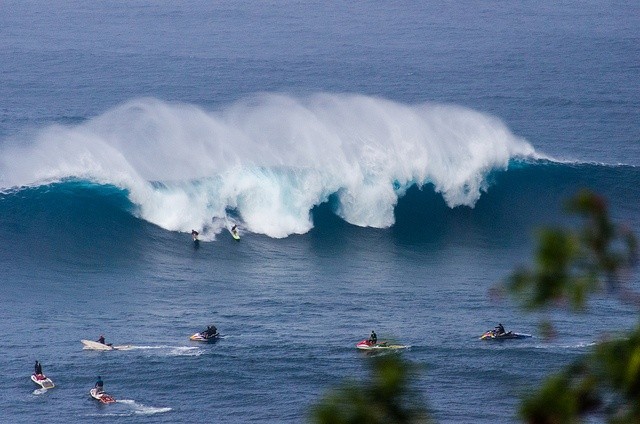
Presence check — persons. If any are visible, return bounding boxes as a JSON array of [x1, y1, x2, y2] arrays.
[[496, 323, 504, 339], [97, 336, 106, 343], [94, 376, 108, 387], [369, 330, 378, 345], [202, 326, 212, 338], [34, 360, 43, 373]]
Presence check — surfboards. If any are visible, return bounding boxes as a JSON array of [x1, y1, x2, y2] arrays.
[[230, 229, 241, 240]]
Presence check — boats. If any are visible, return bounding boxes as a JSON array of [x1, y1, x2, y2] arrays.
[[190, 332, 218, 341], [31, 374, 55, 390], [356, 339, 411, 350], [90, 388, 116, 404], [193, 235, 200, 242], [227, 223, 240, 239], [479, 329, 532, 340]]
